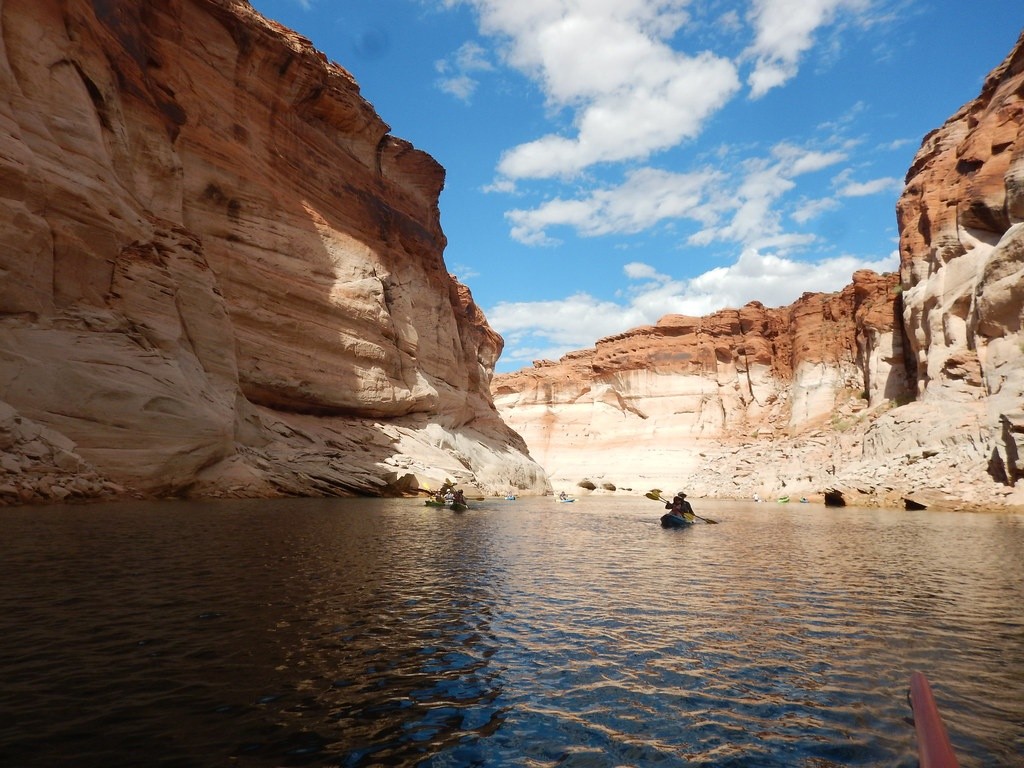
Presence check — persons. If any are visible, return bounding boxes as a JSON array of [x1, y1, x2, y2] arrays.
[[433, 487, 467, 506], [560, 491, 566, 500], [666, 492, 695, 517], [753, 492, 759, 502]]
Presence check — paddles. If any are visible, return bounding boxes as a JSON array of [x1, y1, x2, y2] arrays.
[[652, 490, 694, 521], [646, 493, 717, 524]]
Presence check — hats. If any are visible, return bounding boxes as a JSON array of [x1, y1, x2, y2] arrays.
[[678, 492, 686, 497], [447, 489, 450, 491], [674, 496, 682, 498]]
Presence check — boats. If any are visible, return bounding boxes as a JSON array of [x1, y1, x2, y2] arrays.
[[778, 495, 790, 503], [425, 500, 466, 511], [555, 498, 575, 503], [504, 496, 515, 501], [659, 513, 696, 528], [799, 498, 809, 503]]
